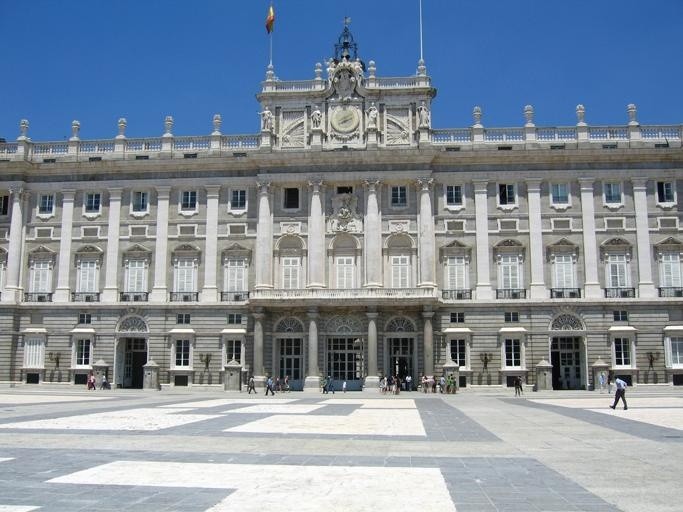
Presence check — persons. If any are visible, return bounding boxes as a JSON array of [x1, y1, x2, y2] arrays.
[[514, 376, 521, 397], [378, 373, 456, 395], [265, 376, 275, 396], [416, 101, 430, 127], [248, 376, 258, 394], [284, 376, 290, 392], [309, 105, 321, 128], [88, 373, 96, 390], [275, 376, 282, 393], [258, 107, 272, 129], [610, 376, 627, 410], [320, 375, 334, 394], [364, 101, 378, 127], [343, 379, 347, 393], [100, 372, 108, 390]]
